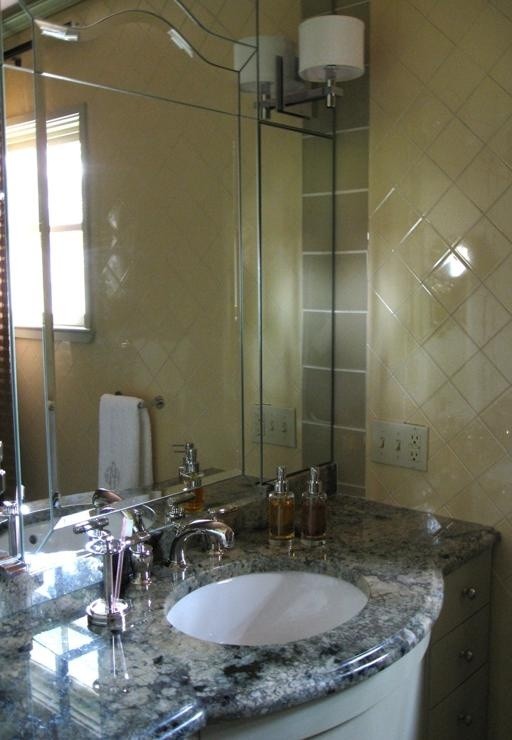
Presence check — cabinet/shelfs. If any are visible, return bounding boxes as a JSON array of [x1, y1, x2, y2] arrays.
[[422, 549, 496, 739]]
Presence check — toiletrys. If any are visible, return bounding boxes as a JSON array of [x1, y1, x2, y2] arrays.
[[300, 466, 328, 540], [174, 442, 204, 511], [267, 467, 296, 539]]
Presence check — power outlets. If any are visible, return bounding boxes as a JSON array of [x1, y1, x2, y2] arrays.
[[401, 422, 430, 472]]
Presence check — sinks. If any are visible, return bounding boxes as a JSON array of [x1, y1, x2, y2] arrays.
[[163, 559, 371, 647], [0, 502, 157, 555]]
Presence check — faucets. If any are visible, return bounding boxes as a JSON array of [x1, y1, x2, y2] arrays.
[[169, 519, 234, 565], [91, 488, 145, 534]]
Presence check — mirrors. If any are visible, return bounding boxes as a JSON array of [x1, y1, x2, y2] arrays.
[[1, 0, 340, 577]]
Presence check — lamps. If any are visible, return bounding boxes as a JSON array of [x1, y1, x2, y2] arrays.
[[233, 32, 281, 122], [282, 13, 366, 114]]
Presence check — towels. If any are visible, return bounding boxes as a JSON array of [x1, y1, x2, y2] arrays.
[[96, 393, 156, 493]]
